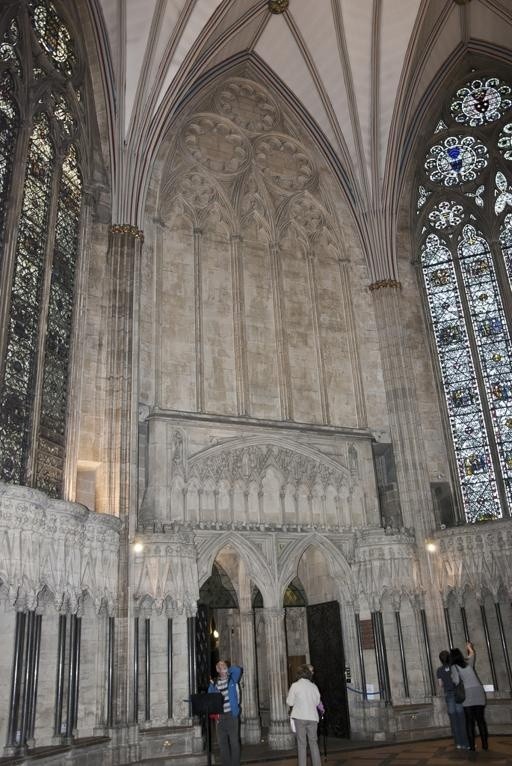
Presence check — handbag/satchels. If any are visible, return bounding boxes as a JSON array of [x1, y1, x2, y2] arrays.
[[453, 680, 466, 705]]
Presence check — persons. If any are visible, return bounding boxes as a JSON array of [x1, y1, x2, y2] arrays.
[[448, 640, 489, 752], [287, 665, 323, 766], [434, 650, 472, 752], [307, 665, 331, 764], [207, 661, 243, 766]]
